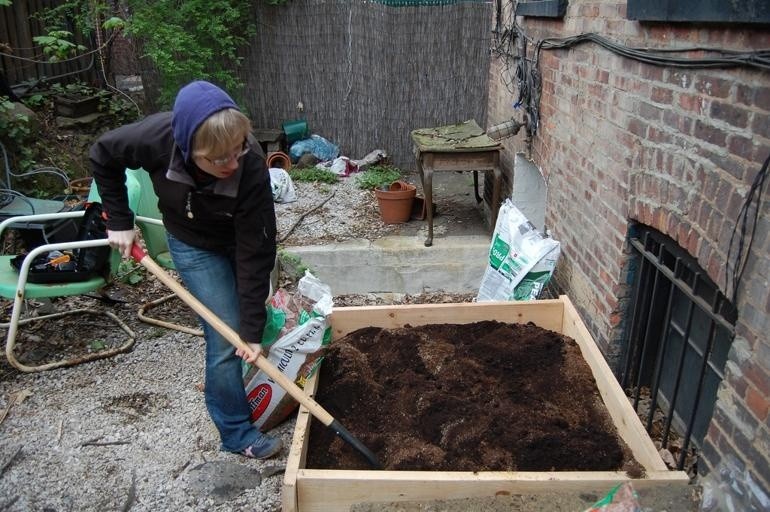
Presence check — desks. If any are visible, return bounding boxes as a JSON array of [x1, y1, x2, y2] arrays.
[[411, 124, 508, 248]]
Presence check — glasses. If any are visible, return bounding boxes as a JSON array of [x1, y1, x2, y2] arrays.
[[204, 141, 252, 166]]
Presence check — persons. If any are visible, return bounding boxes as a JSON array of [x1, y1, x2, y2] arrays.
[[87, 79, 284, 460]]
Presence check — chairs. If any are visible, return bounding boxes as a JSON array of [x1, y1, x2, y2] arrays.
[[125, 168, 204, 335], [0, 143, 78, 255], [1, 171, 141, 373]]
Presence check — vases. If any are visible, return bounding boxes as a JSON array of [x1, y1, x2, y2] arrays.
[[375, 184, 416, 225]]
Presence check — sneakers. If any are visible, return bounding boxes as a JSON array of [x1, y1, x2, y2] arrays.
[[239, 434, 283, 459]]
